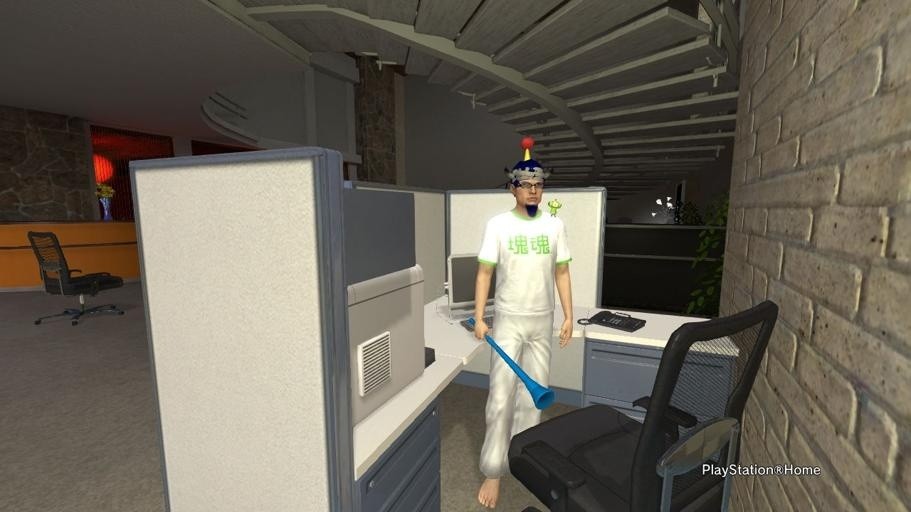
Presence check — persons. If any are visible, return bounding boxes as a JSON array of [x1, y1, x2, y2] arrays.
[[472, 139, 576, 510]]
